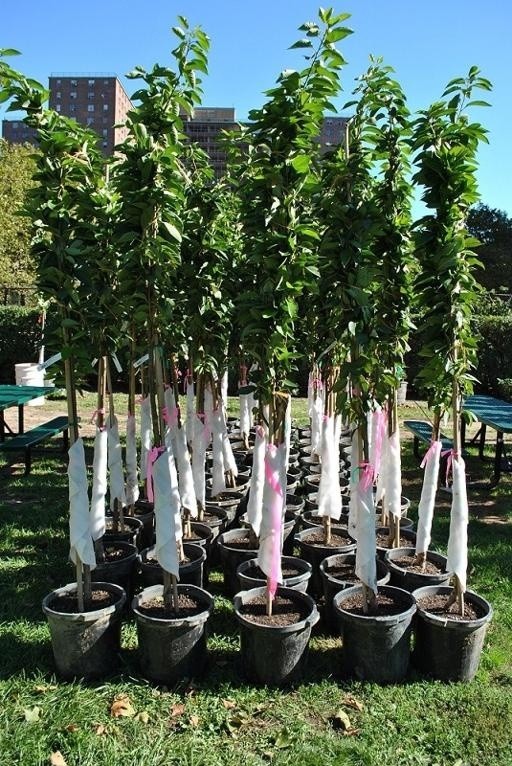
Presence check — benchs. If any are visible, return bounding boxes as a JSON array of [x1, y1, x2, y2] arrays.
[[404, 419, 470, 469], [1, 415, 81, 476]]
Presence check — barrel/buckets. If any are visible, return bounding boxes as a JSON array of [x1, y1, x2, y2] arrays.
[[14, 362, 56, 405]]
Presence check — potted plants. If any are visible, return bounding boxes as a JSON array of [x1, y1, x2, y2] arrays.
[[14, 4, 493, 683]]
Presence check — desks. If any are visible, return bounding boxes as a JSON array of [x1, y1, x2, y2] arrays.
[[1, 384, 57, 440], [461, 395, 512, 475]]
